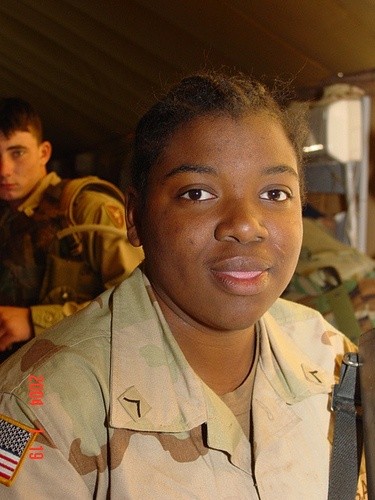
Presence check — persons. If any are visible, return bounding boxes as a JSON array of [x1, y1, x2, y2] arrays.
[[0, 74, 375, 500]]
